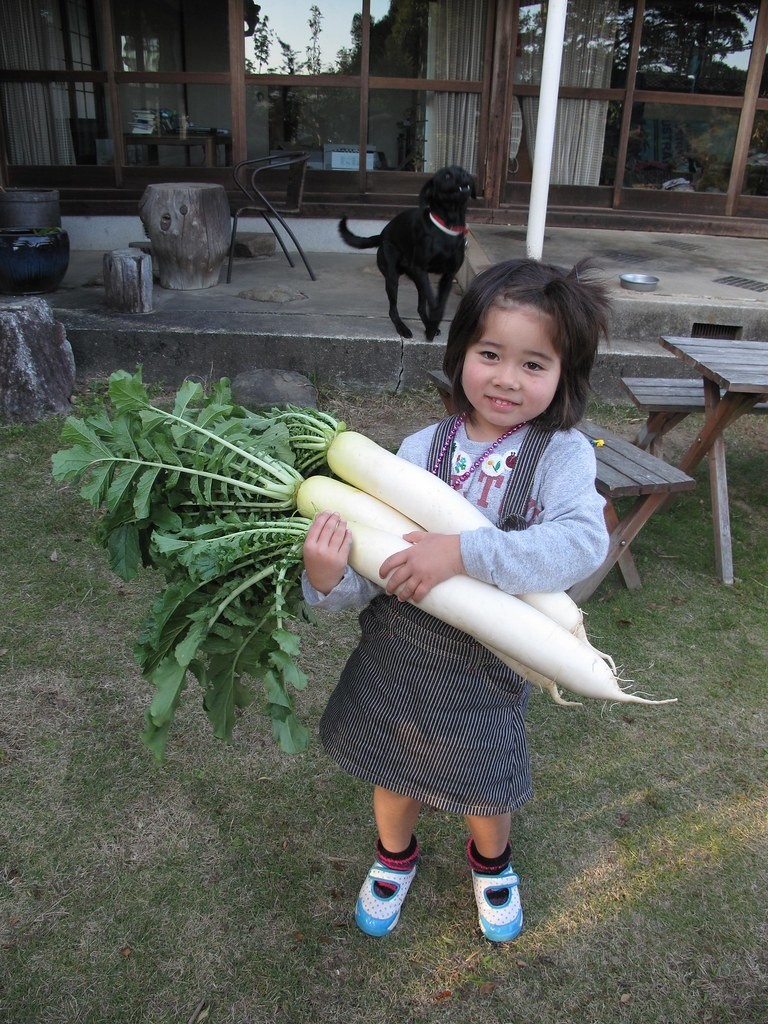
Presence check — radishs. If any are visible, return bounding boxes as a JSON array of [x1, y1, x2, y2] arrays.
[[296, 430, 680, 706]]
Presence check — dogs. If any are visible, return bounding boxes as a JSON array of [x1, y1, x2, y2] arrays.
[[337, 164, 481, 344]]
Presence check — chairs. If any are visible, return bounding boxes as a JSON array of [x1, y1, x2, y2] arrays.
[[225, 151, 317, 281]]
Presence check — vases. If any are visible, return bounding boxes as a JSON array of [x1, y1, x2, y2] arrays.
[[0, 226, 70, 295]]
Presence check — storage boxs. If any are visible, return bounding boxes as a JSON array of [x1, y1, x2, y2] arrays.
[[322, 144, 376, 168]]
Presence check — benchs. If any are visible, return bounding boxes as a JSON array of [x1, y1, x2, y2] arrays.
[[426, 370, 696, 606], [620, 378, 768, 459]]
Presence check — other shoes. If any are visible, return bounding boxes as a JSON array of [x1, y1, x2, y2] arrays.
[[355, 860, 417, 937], [471, 862, 523, 942]]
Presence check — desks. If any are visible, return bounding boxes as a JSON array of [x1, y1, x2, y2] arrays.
[[657, 335, 768, 587], [126, 132, 232, 167]]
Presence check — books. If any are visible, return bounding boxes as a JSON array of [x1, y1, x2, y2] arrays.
[[128, 109, 156, 135]]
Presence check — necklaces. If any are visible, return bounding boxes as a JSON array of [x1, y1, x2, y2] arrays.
[[433, 411, 526, 489]]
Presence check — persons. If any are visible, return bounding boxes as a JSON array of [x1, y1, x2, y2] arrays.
[[302, 259, 613, 943]]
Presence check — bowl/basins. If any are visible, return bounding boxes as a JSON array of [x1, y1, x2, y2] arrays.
[[619, 274, 660, 292]]
